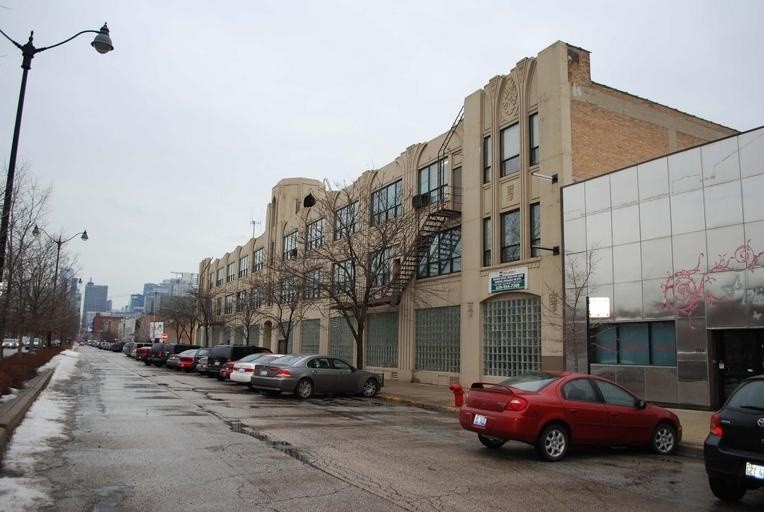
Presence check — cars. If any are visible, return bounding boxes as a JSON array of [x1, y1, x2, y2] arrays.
[[123, 342, 213, 373], [1, 335, 61, 350], [79, 339, 126, 352], [220, 351, 382, 400], [457, 368, 684, 462]]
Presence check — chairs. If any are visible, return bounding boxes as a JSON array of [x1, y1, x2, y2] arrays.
[[317, 360, 329, 368], [568, 388, 586, 399]]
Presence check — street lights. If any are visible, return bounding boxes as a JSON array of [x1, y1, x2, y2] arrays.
[[60, 278, 83, 346], [32, 224, 89, 349], [0, 21, 116, 292]]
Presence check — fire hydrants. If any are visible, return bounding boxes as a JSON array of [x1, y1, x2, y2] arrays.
[[449, 384, 464, 407]]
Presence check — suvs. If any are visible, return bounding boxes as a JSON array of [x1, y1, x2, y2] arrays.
[[703, 373, 764, 504]]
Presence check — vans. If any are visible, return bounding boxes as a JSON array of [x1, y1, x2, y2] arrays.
[[205, 344, 274, 382]]
